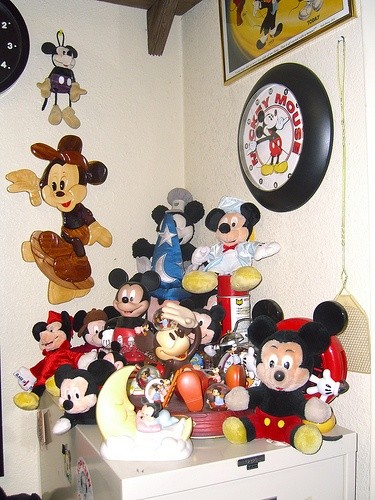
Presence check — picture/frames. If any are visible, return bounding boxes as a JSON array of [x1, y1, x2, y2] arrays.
[[219, 0, 359, 85]]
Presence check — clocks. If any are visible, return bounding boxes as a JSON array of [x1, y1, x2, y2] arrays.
[[0, 1, 30, 92], [238, 62, 333, 212]]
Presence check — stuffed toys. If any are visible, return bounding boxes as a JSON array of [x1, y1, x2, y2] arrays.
[[181, 298, 224, 355], [12, 268, 161, 436], [221, 315, 332, 455], [132, 188, 281, 292]]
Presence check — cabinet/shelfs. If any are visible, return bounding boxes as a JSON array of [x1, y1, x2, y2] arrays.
[[38, 385, 357, 500]]
